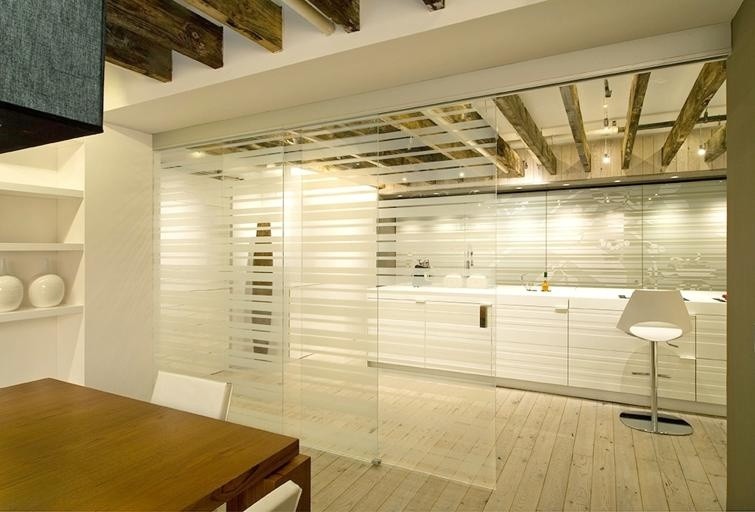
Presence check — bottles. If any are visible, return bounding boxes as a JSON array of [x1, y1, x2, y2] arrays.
[[542, 272, 549, 290]]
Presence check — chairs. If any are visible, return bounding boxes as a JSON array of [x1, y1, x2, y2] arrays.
[[615, 289, 695, 437], [242, 480, 303, 512], [150, 370, 233, 422]]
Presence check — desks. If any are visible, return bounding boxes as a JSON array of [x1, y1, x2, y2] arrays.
[[0, 377, 311, 512]]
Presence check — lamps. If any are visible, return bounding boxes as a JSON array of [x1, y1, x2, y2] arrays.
[[602, 118, 610, 133], [697, 143, 706, 155], [603, 153, 610, 163], [610, 120, 618, 134]]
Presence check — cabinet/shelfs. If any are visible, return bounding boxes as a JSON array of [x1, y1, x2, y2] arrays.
[[0, 137, 84, 324]]
